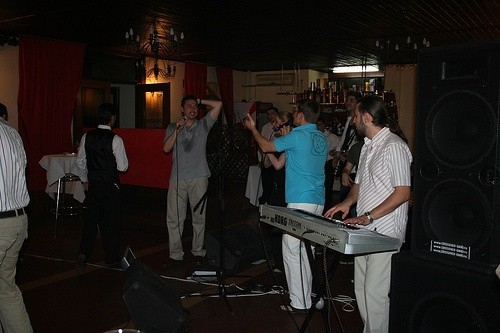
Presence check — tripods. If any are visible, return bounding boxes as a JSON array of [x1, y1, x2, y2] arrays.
[[184, 132, 250, 316]]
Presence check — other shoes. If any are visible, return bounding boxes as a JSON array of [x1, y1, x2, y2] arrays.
[[194, 255, 204, 264], [161, 258, 179, 270]]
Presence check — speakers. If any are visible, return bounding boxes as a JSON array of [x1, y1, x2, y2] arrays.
[[388, 38, 500, 333]]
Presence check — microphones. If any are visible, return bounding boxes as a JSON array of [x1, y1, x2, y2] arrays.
[[274, 118, 293, 132], [177, 115, 189, 130]]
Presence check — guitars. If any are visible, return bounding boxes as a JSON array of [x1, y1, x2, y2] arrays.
[[334, 149, 346, 177]]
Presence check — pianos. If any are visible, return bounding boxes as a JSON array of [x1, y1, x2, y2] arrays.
[[258, 201, 400, 256]]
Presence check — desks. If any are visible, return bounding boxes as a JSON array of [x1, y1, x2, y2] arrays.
[[38, 153, 89, 222]]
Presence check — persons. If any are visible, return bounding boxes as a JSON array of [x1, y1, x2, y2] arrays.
[[247, 92, 412, 333], [76, 104, 128, 260], [162, 97, 222, 266], [0, 102, 39, 333]]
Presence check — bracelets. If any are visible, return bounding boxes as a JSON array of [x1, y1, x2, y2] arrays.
[[200, 98, 201, 105]]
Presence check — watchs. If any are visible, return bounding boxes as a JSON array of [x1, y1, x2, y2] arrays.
[[364, 211, 374, 225]]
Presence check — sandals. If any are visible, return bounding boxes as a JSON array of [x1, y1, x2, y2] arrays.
[[279, 303, 308, 312]]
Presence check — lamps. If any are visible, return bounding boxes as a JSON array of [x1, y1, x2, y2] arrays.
[[124, 8, 187, 79]]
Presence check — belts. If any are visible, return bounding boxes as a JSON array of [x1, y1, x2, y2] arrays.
[[0, 207, 24, 219]]
